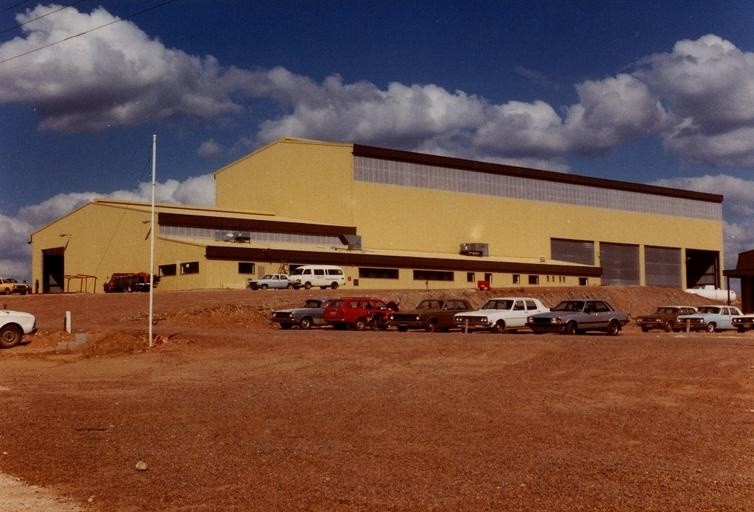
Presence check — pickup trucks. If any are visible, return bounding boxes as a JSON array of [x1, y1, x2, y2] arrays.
[[103, 272, 160, 293]]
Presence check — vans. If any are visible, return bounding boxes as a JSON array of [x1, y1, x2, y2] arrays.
[[289, 264, 346, 289]]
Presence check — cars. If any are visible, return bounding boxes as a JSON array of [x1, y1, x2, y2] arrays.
[[249, 273, 290, 289], [0, 309, 37, 348], [0, 278, 29, 296], [635, 304, 754, 332]]
